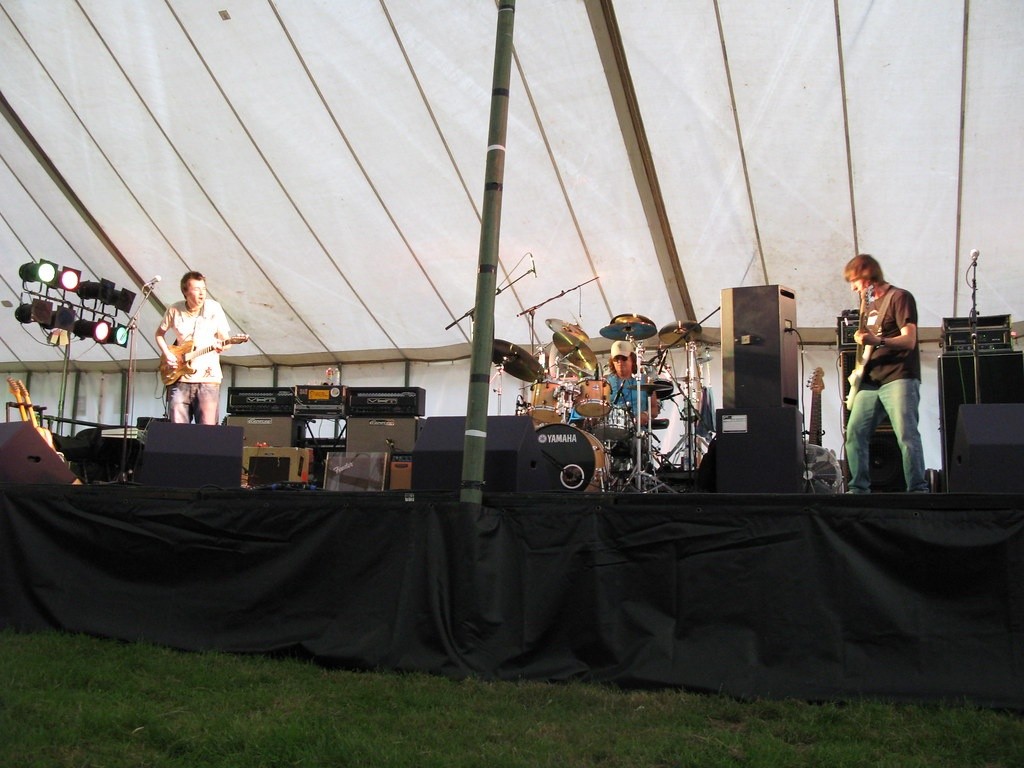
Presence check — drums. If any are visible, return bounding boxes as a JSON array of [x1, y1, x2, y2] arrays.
[[527, 378, 567, 424], [574, 375, 612, 418], [583, 405, 633, 442], [518, 423, 611, 492]]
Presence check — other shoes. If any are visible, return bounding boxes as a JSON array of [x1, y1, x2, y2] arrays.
[[911, 487, 929, 493], [846, 487, 871, 494]]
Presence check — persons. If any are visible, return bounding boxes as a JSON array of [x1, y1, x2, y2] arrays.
[[608, 340, 658, 486], [843, 254, 931, 493], [155, 271, 231, 425]]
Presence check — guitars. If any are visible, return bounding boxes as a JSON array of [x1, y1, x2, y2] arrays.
[[158, 334, 251, 386], [846, 290, 869, 411], [5, 375, 58, 454], [806, 366, 828, 446]]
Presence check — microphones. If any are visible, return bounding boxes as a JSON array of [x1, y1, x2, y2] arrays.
[[143, 275, 162, 286], [594, 364, 599, 381], [521, 396, 528, 409], [562, 470, 574, 479], [530, 254, 537, 278], [970, 249, 979, 260], [613, 379, 626, 404]]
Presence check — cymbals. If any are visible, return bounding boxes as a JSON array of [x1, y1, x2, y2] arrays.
[[553, 330, 599, 371], [609, 313, 658, 327], [598, 322, 658, 341], [491, 338, 545, 383], [625, 383, 673, 391], [658, 321, 702, 347], [545, 317, 590, 345]]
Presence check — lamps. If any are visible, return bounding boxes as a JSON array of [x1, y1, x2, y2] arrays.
[[15, 260, 137, 347]]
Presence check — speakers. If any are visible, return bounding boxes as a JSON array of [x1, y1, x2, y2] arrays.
[[839, 351, 910, 492], [142, 415, 542, 493], [939, 350, 1024, 495], [716, 284, 808, 494], [0, 421, 78, 483]]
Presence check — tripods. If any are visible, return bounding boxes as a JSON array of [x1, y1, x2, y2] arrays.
[[621, 332, 710, 494]]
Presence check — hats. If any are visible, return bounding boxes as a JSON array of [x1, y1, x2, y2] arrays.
[[611, 340, 636, 359]]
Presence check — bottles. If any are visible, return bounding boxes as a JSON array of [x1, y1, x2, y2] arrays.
[[683, 445, 694, 470], [326, 368, 333, 385], [333, 367, 340, 386]]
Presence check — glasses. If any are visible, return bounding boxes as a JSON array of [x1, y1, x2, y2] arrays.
[[613, 356, 632, 362]]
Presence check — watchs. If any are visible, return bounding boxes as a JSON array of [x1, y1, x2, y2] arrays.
[[877, 337, 885, 349]]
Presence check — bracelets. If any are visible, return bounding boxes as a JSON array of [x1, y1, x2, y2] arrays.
[[643, 411, 648, 416]]
